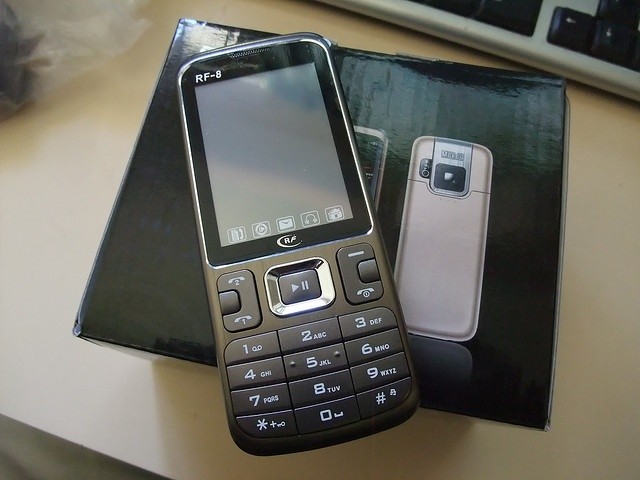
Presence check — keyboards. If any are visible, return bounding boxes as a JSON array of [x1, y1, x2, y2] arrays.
[[326, 0, 640, 104]]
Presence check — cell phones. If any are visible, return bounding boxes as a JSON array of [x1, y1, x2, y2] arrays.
[[350, 121, 389, 223], [176, 29, 422, 457], [392, 134, 496, 344]]
